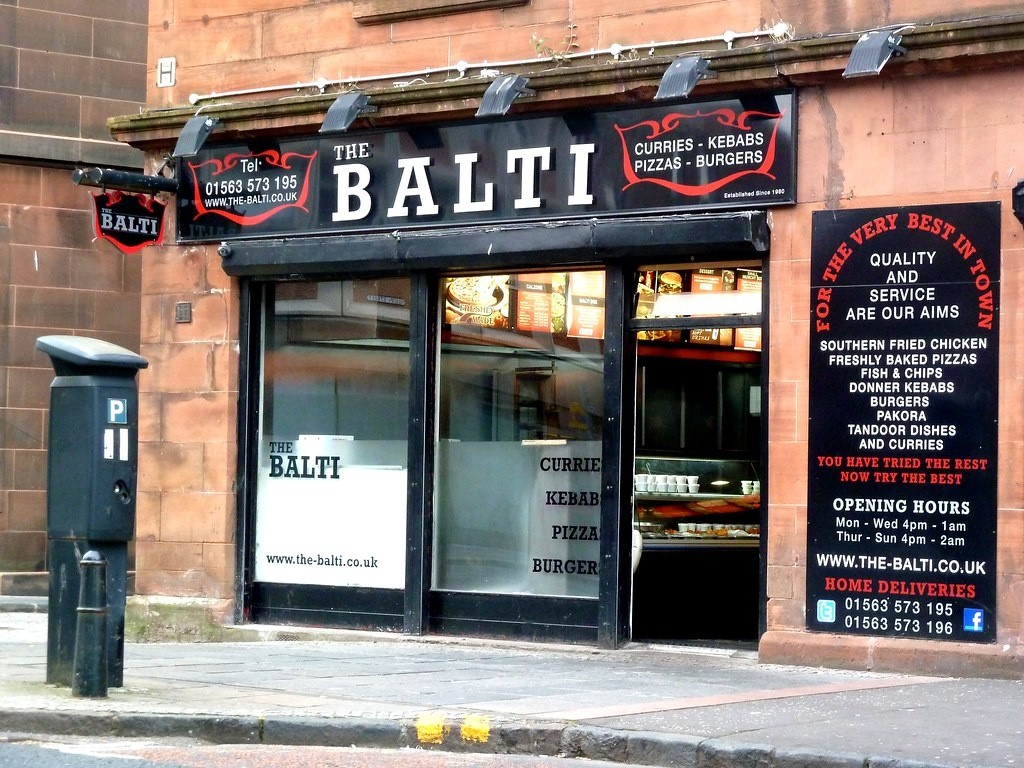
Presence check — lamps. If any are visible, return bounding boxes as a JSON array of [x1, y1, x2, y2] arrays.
[[841, 30, 907, 79], [172, 115, 219, 157], [475, 75, 536, 117], [652, 56, 717, 101], [318, 93, 378, 134]]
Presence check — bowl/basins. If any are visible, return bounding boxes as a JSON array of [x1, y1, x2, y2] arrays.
[[634, 474, 700, 493], [678, 523, 760, 533], [741, 481, 760, 495], [634, 522, 662, 532]]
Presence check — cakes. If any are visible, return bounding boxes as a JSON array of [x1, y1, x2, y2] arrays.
[[447, 277, 497, 307], [551, 292, 565, 317]]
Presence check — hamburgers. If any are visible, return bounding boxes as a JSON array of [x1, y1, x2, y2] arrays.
[[657, 272, 682, 295]]
